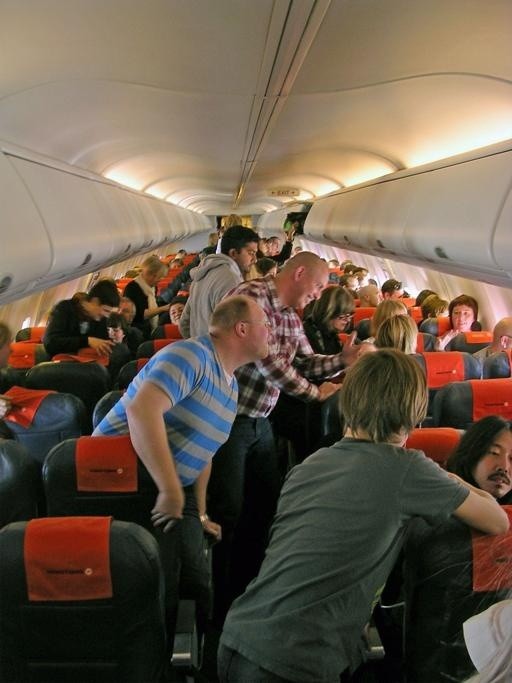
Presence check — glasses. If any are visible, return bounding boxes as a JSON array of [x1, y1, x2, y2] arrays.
[[333, 310, 356, 321]]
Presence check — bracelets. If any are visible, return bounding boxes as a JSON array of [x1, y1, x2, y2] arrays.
[[196, 512, 211, 525]]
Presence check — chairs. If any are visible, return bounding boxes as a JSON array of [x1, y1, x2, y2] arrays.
[[0, 247, 209, 680], [283, 247, 510, 681]]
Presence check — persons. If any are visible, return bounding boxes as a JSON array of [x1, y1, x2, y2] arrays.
[[122, 259, 170, 340], [472, 317, 509, 362], [416, 294, 449, 333], [106, 313, 127, 344], [0, 313, 20, 434], [357, 285, 380, 307], [169, 301, 186, 325], [355, 300, 407, 345], [44, 279, 120, 357], [216, 345, 510, 681], [202, 232, 219, 255], [435, 294, 492, 352], [216, 348, 510, 681], [168, 249, 187, 269], [327, 259, 377, 292], [415, 290, 437, 306], [372, 410, 512, 640], [377, 280, 403, 307], [244, 225, 296, 282], [201, 251, 378, 529], [449, 594, 511, 681], [270, 285, 355, 458], [179, 226, 259, 340], [88, 291, 274, 679], [217, 214, 242, 253], [201, 248, 378, 529], [374, 315, 418, 354]]
